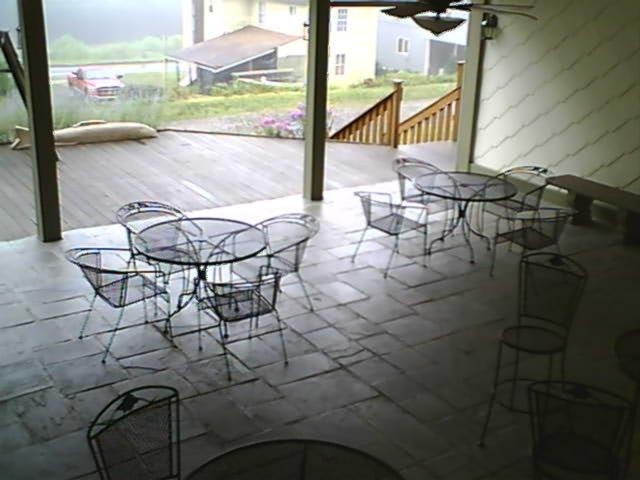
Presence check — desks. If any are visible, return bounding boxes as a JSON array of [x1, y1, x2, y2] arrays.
[[609, 329, 640, 461], [133, 217, 269, 337], [185, 439, 406, 480]]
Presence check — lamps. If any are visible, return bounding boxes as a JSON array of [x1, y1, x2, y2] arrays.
[[412, 11, 466, 35], [480, 12, 498, 40]]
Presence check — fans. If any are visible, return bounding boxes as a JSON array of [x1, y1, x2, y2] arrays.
[[330, 0, 539, 22]]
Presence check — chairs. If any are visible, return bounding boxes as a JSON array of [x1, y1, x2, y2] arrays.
[[521, 380, 634, 480], [85, 384, 183, 479], [349, 154, 572, 281], [63, 246, 174, 366], [111, 200, 209, 326], [211, 211, 321, 328], [477, 250, 589, 447], [191, 263, 290, 381]]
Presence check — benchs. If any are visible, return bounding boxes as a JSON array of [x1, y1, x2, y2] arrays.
[[545, 170, 640, 247]]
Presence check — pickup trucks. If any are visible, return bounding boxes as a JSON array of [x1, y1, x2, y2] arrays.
[[67, 66, 126, 102]]
